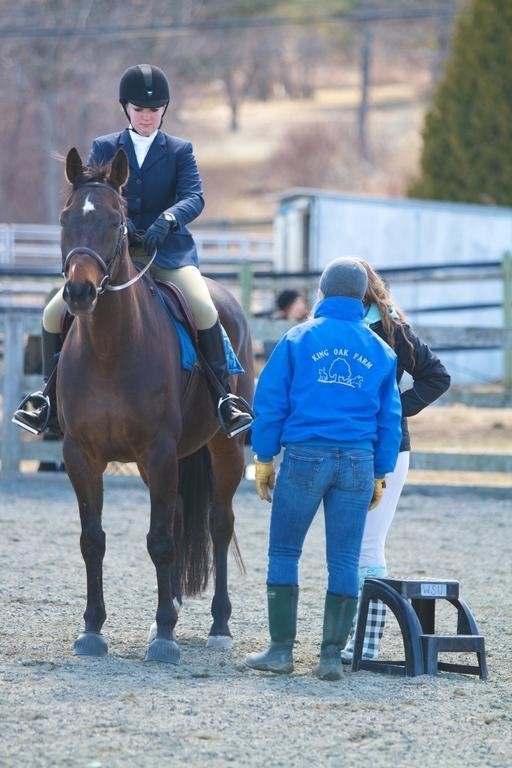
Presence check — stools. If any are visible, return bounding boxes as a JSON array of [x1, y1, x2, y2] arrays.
[[352, 576, 489, 680]]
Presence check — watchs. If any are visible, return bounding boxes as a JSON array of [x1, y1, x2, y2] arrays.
[[163, 213, 174, 222]]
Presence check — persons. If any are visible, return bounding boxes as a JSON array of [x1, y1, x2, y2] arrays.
[[265, 284, 313, 362], [9, 59, 256, 441], [342, 259, 452, 669], [23, 286, 71, 475], [243, 258, 406, 682]]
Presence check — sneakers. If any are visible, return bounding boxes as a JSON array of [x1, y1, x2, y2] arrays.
[[333, 645, 376, 663]]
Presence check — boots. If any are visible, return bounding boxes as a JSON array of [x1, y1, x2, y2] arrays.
[[245, 583, 298, 674], [14, 327, 63, 434], [196, 316, 252, 431], [312, 594, 358, 681]]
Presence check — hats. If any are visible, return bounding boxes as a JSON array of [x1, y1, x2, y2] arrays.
[[319, 258, 367, 300], [278, 290, 300, 311]]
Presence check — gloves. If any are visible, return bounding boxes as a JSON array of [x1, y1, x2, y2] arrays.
[[253, 454, 276, 502], [143, 215, 174, 250], [368, 478, 385, 510], [127, 217, 142, 247]]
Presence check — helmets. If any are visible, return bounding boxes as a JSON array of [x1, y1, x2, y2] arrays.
[[119, 63, 170, 108]]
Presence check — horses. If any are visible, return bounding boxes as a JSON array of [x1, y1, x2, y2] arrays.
[[53, 145, 256, 665]]
